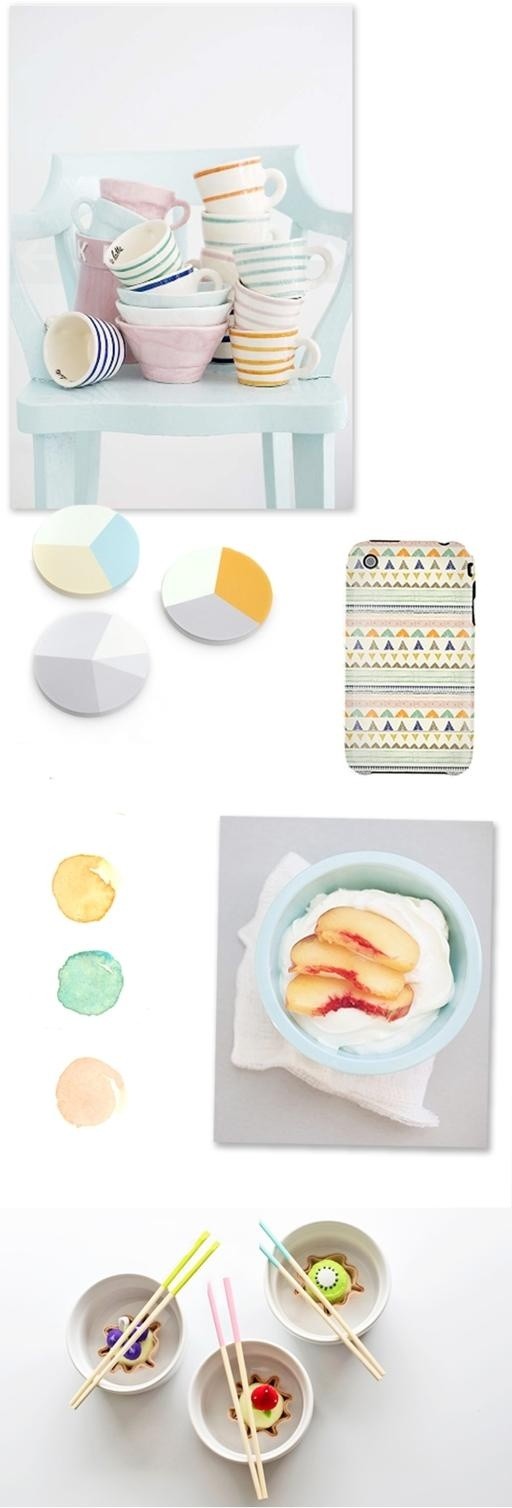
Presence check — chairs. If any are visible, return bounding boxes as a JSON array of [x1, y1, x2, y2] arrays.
[[12, 146, 353, 508]]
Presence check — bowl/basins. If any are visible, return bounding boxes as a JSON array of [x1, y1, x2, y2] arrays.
[[262, 1220, 390, 1337], [190, 1343, 316, 1464], [108, 286, 233, 389], [68, 1275, 185, 1396], [252, 851, 486, 1079]]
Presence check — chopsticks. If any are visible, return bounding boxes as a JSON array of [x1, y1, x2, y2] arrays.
[[209, 1276, 276, 1504], [71, 1230, 220, 1411], [252, 1228, 390, 1390]]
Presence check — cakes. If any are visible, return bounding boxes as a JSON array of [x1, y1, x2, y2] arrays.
[[296, 1251, 364, 1312], [277, 885, 456, 1064], [99, 1312, 164, 1374], [228, 1370, 293, 1438]]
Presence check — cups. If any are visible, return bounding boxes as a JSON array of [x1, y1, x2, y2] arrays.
[[42, 306, 122, 389], [196, 161, 339, 394], [72, 176, 193, 314]]
